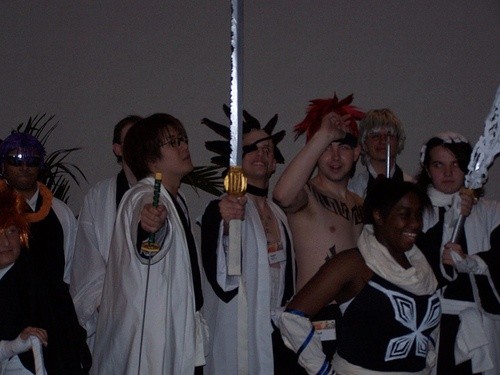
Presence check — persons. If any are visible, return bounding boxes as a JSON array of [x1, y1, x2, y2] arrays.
[[0, 131, 93, 375], [194, 104, 298, 375], [271, 93, 500, 375], [68, 115, 145, 357], [88, 112, 210, 375], [277, 180, 442, 375]]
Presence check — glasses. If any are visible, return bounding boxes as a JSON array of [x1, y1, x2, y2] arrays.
[[160, 135, 188, 148], [3, 155, 44, 168]]
